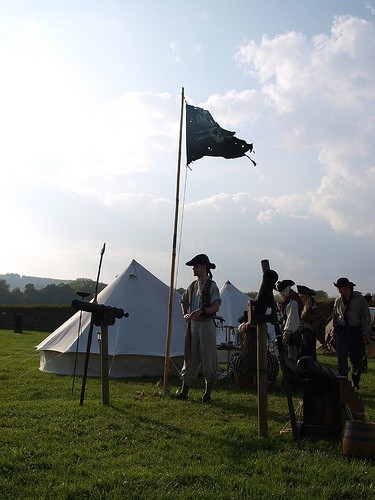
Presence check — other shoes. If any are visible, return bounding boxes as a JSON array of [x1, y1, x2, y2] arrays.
[[351, 381, 359, 391]]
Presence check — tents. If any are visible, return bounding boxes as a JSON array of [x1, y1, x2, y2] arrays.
[[34, 259, 186, 378], [214, 279, 281, 345]]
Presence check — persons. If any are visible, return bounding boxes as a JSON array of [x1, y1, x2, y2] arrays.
[[228, 311, 279, 389], [333, 278, 372, 389], [273, 280, 304, 391], [297, 285, 327, 361], [173, 254, 221, 404]]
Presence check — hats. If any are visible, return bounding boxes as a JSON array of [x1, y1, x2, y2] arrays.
[[333, 278, 356, 288], [296, 285, 317, 296], [186, 254, 215, 269], [272, 280, 295, 292]]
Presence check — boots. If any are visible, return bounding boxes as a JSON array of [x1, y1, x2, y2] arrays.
[[199, 380, 213, 401], [171, 381, 189, 400]]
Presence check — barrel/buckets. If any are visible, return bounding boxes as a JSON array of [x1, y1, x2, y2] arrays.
[[342, 419, 375, 466]]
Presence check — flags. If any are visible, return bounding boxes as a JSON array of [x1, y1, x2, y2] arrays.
[[184, 104, 258, 170]]
